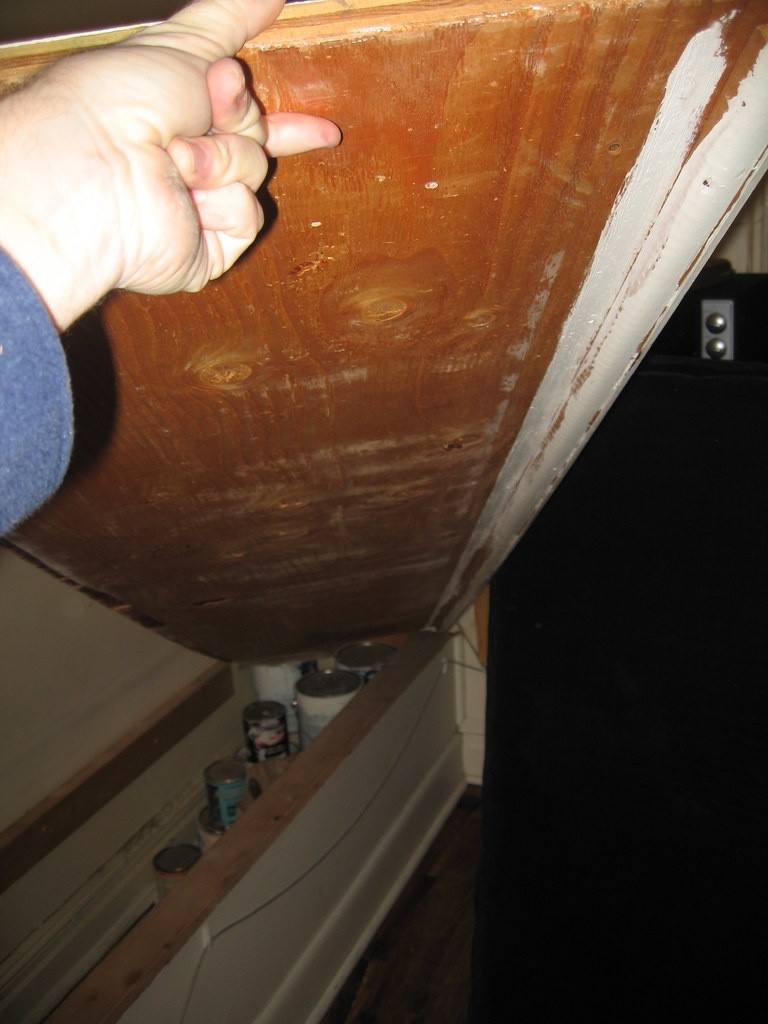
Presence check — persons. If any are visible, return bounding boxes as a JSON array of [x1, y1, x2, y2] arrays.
[[0, 0, 344, 539]]
